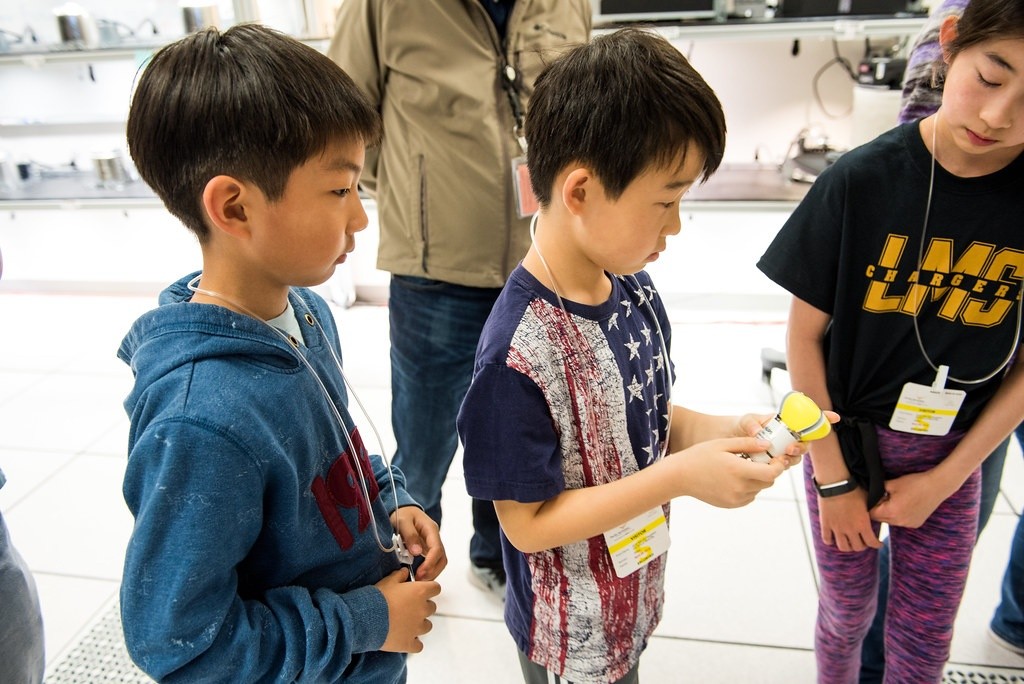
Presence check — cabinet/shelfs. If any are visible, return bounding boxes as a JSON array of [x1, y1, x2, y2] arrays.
[[0, 0, 388, 307], [587, 0, 929, 323]]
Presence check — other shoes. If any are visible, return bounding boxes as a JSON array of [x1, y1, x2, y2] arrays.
[[467, 561, 511, 607], [991, 621, 1024, 655]]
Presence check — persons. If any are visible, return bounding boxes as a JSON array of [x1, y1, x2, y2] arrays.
[[756, 0, 1024, 684], [0, 468, 46, 684], [322, 0, 594, 607], [116, 24, 449, 684], [453, 29, 841, 684]]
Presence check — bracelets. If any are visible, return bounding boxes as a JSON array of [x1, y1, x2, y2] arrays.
[[812, 476, 858, 498]]
